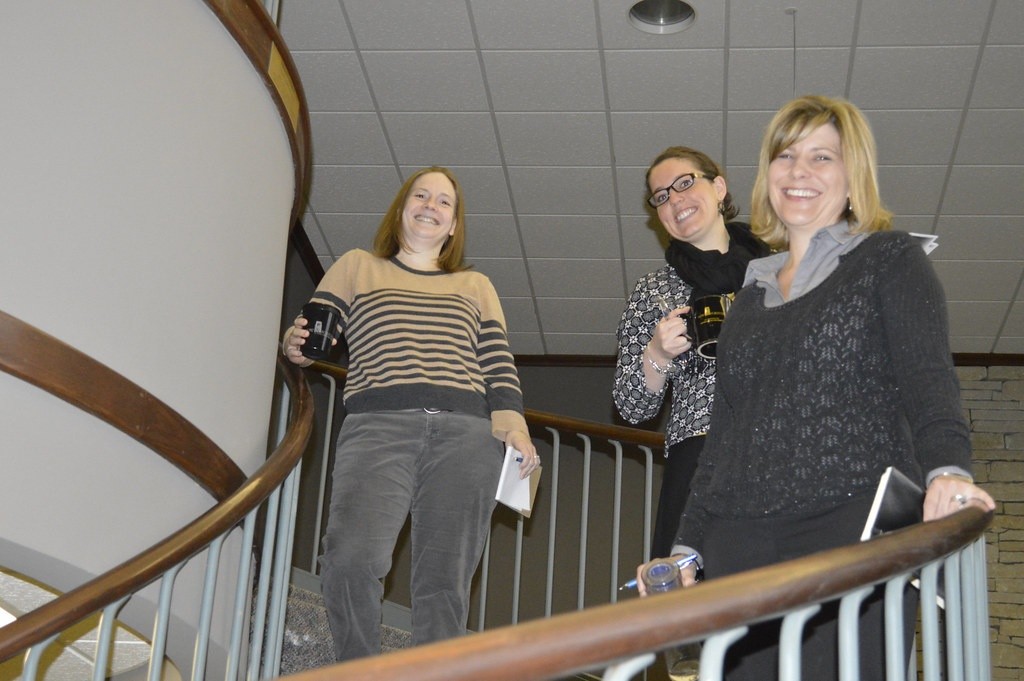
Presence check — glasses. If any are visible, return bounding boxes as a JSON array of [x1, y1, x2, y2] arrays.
[[647, 172, 713, 209]]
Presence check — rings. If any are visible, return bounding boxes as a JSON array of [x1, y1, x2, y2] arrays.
[[533, 454, 540, 459], [950, 493, 966, 506]]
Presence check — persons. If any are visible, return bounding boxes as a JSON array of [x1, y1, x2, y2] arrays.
[[612, 94, 997, 681], [280, 165, 541, 664]]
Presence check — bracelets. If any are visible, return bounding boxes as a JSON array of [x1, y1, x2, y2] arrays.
[[646, 342, 674, 374], [926, 471, 974, 485]]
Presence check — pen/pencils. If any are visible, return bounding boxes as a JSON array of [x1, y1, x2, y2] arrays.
[[618, 555, 697, 593], [663, 310, 687, 363], [515, 458, 524, 463]]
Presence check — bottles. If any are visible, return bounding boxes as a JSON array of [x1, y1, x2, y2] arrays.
[[641, 557, 710, 681]]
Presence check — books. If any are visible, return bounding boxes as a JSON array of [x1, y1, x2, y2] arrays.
[[494, 446, 543, 519], [860, 466, 945, 608]]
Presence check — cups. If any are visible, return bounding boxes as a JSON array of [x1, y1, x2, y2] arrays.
[[300, 302, 342, 358], [676, 294, 733, 358]]
